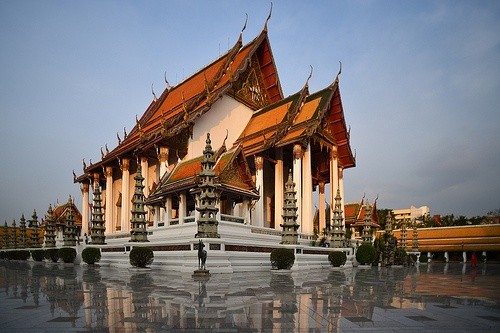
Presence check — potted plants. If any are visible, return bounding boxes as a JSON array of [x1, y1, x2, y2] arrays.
[[31, 249, 46, 264], [127, 246, 155, 272], [269, 247, 295, 274], [328, 250, 348, 271], [355, 243, 378, 270], [0, 250, 31, 263], [44, 248, 61, 265], [387, 248, 418, 269], [81, 247, 102, 269], [57, 247, 78, 265]]
